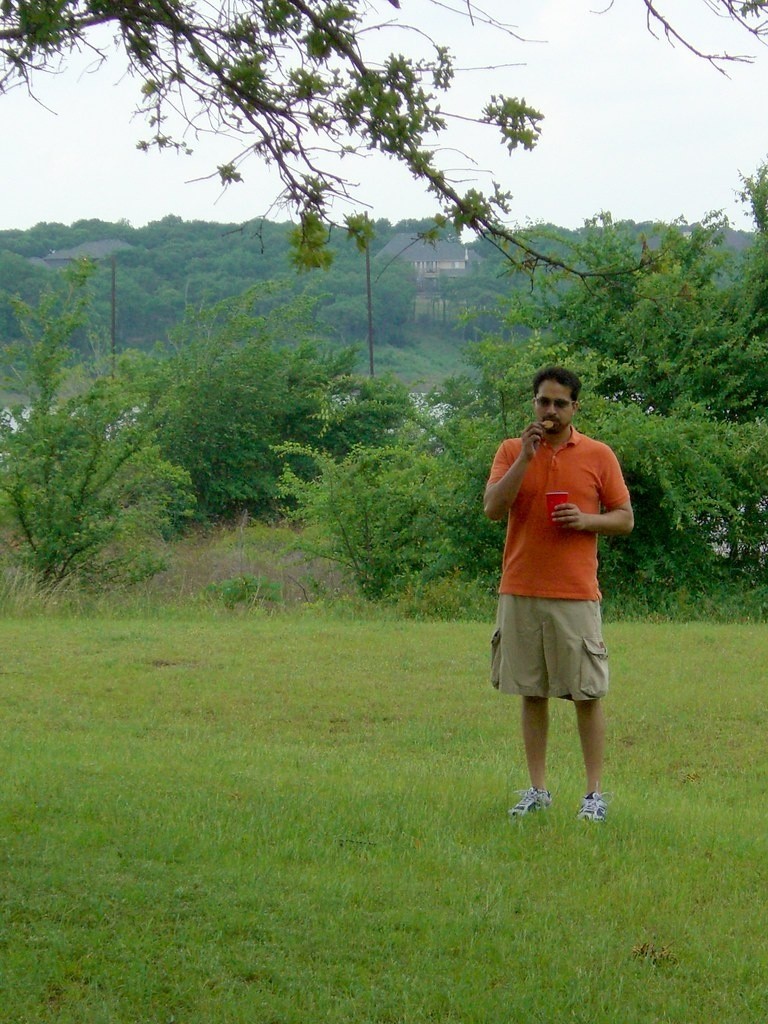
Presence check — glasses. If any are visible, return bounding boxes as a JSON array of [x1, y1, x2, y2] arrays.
[[535, 397, 575, 409]]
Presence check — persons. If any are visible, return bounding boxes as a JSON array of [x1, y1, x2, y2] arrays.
[[483, 367, 634, 821]]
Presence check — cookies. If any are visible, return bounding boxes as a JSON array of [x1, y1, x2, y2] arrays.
[[542, 420, 554, 428]]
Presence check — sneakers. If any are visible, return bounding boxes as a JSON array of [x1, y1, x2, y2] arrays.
[[508, 785, 551, 816], [577, 781, 613, 822]]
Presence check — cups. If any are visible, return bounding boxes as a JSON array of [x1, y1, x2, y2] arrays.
[[544, 492, 569, 527]]
